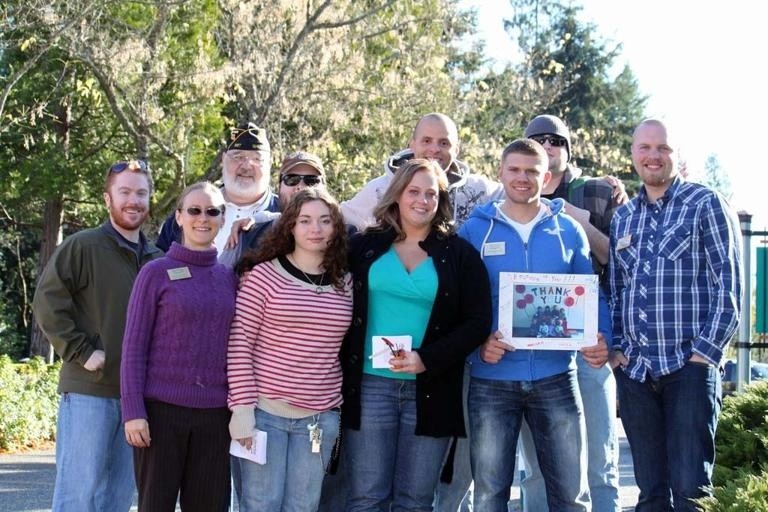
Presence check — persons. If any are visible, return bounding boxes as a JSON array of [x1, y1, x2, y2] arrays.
[[606, 120, 742, 512], [225, 110, 508, 252], [121, 180, 239, 512], [217, 152, 352, 269], [34, 162, 166, 512], [228, 187, 355, 512], [456, 138, 612, 512], [524, 115, 630, 299], [333, 159, 493, 512], [157, 121, 283, 252]]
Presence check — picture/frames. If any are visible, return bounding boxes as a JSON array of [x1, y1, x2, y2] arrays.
[[497, 273, 598, 351]]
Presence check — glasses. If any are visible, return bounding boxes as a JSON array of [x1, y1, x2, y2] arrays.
[[180, 206, 224, 217], [531, 134, 566, 147], [280, 173, 321, 186], [110, 160, 147, 173]]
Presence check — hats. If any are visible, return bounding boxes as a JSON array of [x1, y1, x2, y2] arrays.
[[277, 151, 327, 178], [524, 113, 571, 163], [223, 121, 272, 152]]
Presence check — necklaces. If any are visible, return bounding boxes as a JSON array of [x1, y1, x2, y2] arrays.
[[291, 255, 325, 294]]
